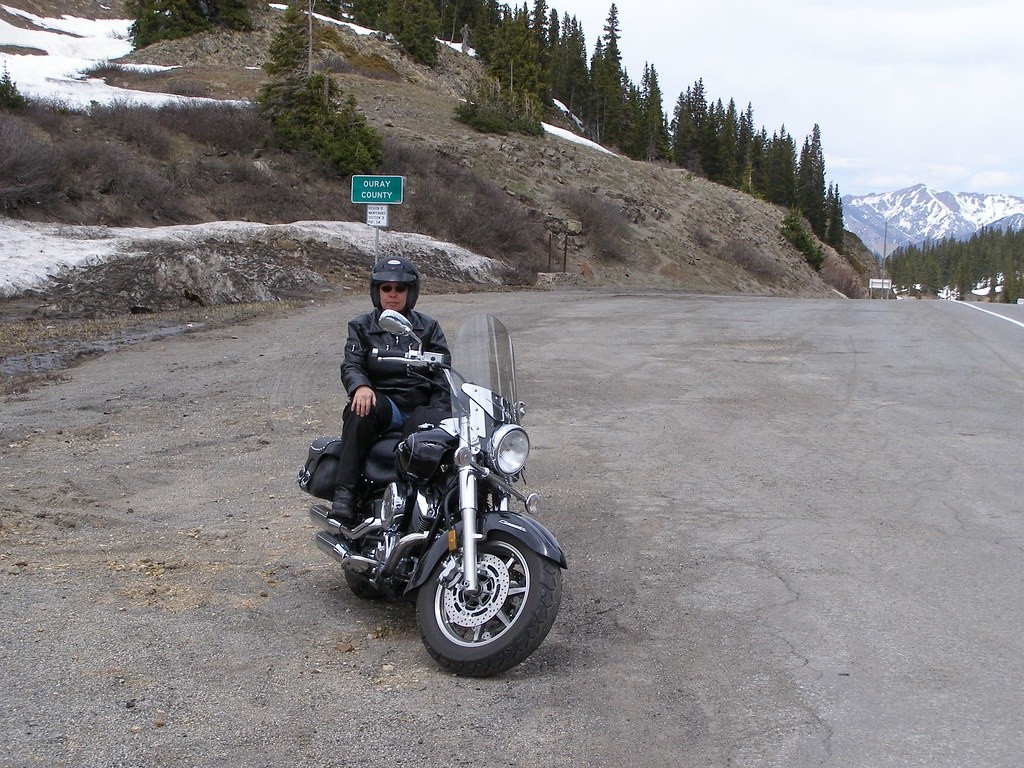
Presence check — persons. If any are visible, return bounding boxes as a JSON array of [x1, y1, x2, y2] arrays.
[[329, 255, 452, 521]]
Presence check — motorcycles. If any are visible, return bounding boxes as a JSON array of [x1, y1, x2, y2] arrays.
[[297, 311, 568, 676]]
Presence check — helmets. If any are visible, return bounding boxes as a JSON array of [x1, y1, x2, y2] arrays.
[[370, 256, 419, 309]]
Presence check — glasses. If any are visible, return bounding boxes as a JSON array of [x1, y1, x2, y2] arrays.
[[379, 285, 408, 292]]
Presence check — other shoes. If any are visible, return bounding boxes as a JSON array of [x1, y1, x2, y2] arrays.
[[332, 490, 357, 522]]
